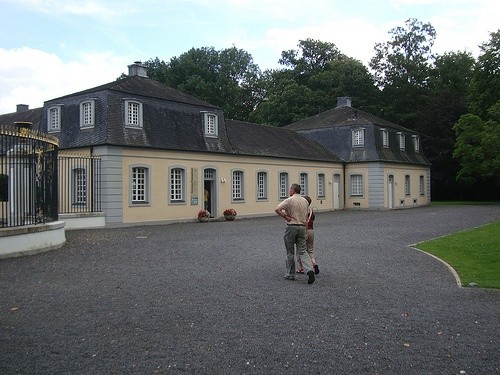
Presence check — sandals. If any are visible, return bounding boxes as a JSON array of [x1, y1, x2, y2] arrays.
[[296, 269, 304, 274], [284, 275, 295, 281], [308, 271, 315, 284], [313, 263, 319, 275]]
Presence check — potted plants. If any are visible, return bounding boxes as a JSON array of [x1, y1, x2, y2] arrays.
[[198, 210, 210, 221], [223, 208, 237, 221]]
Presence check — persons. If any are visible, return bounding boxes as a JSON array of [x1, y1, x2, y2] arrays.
[[294, 196, 320, 275], [275, 184, 315, 285], [204, 187, 209, 210]]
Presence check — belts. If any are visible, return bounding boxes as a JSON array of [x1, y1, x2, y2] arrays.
[[287, 223, 306, 226]]
